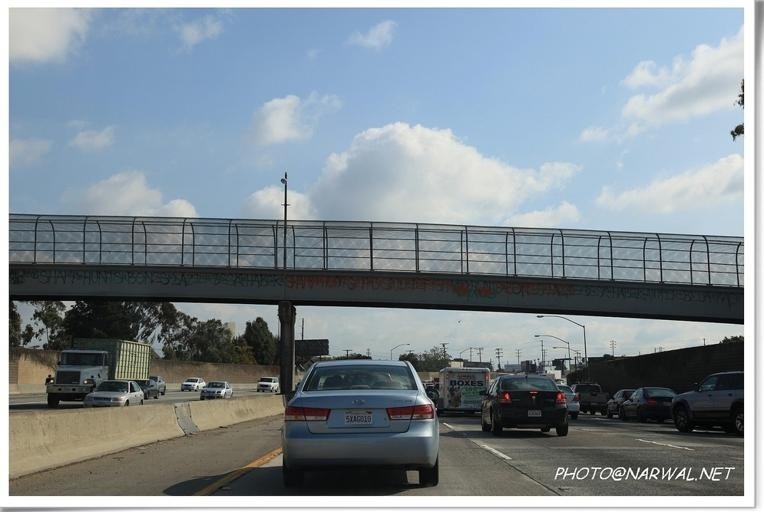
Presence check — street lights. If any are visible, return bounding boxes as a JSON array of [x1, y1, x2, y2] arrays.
[[459, 347, 472, 367], [390, 343, 411, 361], [533, 314, 588, 369], [280, 171, 291, 269]]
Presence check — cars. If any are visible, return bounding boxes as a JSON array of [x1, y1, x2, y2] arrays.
[[137, 378, 159, 400], [150, 375, 166, 395], [83, 380, 143, 408], [422, 366, 675, 437], [181, 377, 205, 391], [200, 381, 234, 400], [281, 359, 440, 491]]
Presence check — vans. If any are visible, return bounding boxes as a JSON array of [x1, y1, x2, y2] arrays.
[[256, 376, 279, 393]]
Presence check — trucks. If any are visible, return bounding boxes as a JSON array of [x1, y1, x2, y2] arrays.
[[42, 336, 153, 409]]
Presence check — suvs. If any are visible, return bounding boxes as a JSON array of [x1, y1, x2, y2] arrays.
[[670, 369, 744, 436]]
